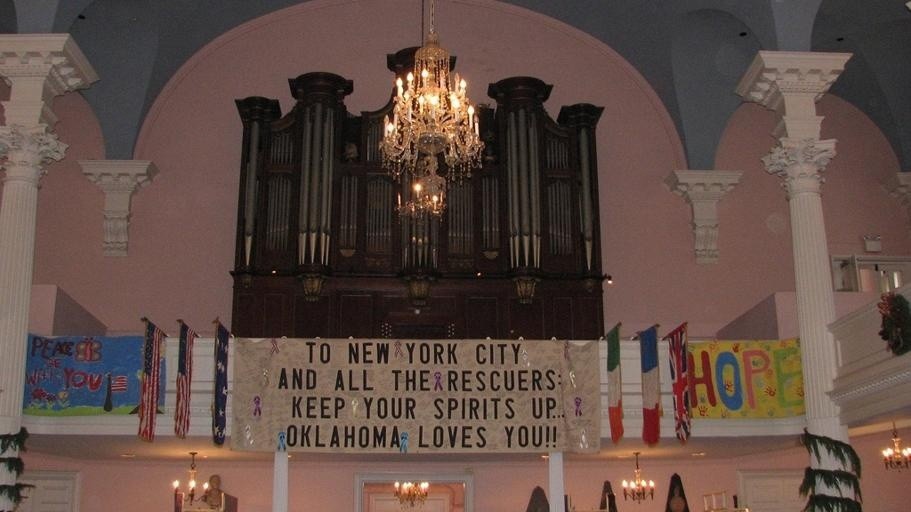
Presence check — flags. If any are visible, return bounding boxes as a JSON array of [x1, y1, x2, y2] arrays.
[[138, 324, 228, 446], [607, 326, 690, 447], [110, 375, 128, 393]]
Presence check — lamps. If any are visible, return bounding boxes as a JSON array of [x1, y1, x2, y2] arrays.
[[172, 452, 209, 505], [394, 481, 431, 510], [395, 153, 447, 222], [880, 417, 911, 473], [379, 0, 486, 180], [621, 452, 655, 504], [863, 232, 882, 252]]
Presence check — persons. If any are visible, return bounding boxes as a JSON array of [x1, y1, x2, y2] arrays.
[[203, 474, 221, 512]]
